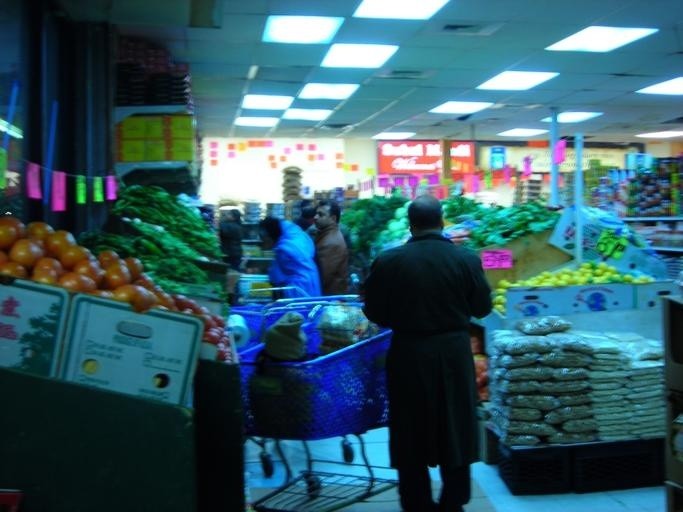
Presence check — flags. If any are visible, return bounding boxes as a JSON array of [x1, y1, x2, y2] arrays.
[[209, 134, 566, 201], [0, 148, 117, 213]]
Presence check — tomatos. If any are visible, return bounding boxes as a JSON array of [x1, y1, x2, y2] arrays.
[[0, 215, 233, 363]]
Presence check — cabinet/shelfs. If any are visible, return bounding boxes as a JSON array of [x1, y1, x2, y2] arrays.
[[348, 217, 682, 501], [1, 215, 252, 512]]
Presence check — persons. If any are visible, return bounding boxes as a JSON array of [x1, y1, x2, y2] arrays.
[[360, 194, 494, 512], [218, 197, 350, 309]]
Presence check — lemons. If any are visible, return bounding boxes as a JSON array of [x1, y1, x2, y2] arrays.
[[493, 262, 655, 316]]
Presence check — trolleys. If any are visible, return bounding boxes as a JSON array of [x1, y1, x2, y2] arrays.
[[225, 284, 392, 495]]
